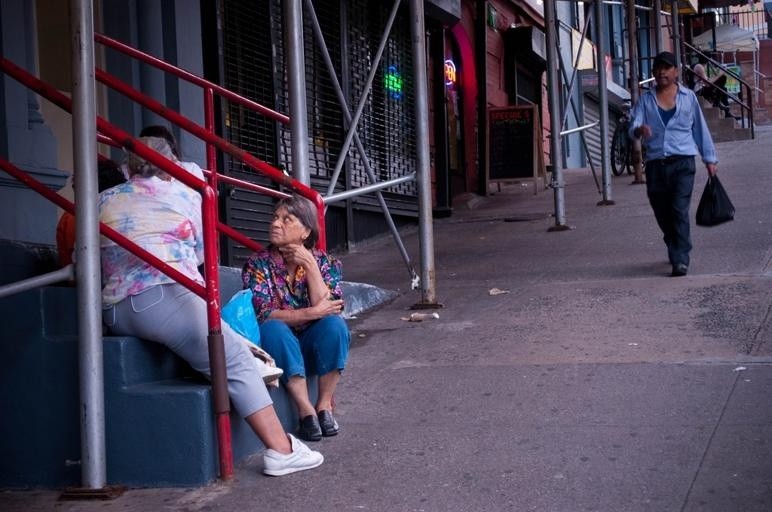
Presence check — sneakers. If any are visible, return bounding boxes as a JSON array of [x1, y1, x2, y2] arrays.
[[255, 356, 284, 384], [263, 432, 325, 476]]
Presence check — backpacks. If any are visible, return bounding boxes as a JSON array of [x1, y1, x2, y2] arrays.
[[685, 63, 700, 89]]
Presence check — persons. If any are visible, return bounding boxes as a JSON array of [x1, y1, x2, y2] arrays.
[[242, 194, 350, 441], [694, 51, 741, 120], [628, 52, 719, 276], [56, 126, 324, 476], [763, 0, 772, 38]]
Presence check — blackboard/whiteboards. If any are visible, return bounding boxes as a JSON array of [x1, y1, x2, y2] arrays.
[[486, 105, 538, 182]]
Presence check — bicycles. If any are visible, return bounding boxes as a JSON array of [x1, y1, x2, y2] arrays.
[[611, 99, 645, 176]]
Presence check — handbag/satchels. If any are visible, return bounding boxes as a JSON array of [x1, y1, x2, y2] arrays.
[[220, 287, 262, 348]]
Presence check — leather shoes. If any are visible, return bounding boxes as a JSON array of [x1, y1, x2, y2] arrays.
[[713, 100, 730, 111], [317, 409, 339, 436], [300, 414, 323, 441], [725, 111, 741, 120], [671, 261, 687, 275]]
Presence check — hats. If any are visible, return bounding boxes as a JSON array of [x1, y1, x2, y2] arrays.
[[653, 51, 677, 69]]
